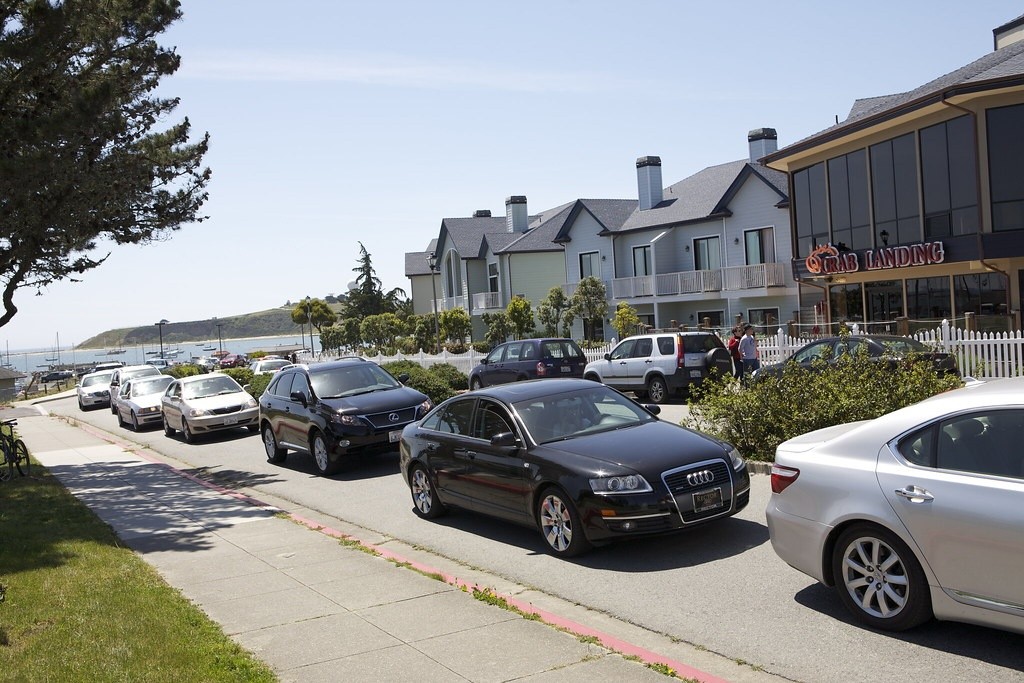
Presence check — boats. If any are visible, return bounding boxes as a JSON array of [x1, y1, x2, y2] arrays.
[[145, 340, 184, 359], [203, 338, 217, 351], [38, 365, 51, 367], [196, 344, 204, 346], [107, 342, 127, 354], [0, 339, 27, 374], [46, 352, 58, 361]]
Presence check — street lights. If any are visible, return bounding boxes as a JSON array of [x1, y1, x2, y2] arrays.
[[216, 324, 222, 361], [425, 252, 442, 355], [155, 323, 166, 359], [306, 296, 314, 358]]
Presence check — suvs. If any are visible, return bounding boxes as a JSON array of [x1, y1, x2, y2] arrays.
[[582, 332, 734, 404], [258, 356, 434, 478]]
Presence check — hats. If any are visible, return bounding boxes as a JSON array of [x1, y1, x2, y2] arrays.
[[743, 324, 752, 332]]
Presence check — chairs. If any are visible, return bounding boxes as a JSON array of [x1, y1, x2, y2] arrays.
[[641, 345, 651, 356], [921, 419, 985, 471], [663, 344, 673, 354]]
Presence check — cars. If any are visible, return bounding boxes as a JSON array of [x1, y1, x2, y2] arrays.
[[766, 375, 1024, 632], [400, 377, 752, 558], [749, 336, 961, 387], [38, 354, 295, 444]]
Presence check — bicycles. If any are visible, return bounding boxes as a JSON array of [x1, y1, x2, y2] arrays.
[[0, 419, 31, 482]]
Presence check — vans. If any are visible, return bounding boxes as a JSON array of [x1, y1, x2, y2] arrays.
[[467, 338, 588, 391]]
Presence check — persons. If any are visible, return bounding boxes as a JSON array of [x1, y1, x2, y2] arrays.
[[729, 322, 760, 386], [292, 351, 296, 364], [284, 352, 289, 360], [552, 398, 591, 437]]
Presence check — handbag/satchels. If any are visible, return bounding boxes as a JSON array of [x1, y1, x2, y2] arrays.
[[731, 346, 736, 356]]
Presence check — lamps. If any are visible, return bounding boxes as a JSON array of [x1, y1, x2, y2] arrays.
[[685, 246, 689, 253], [606, 319, 609, 324], [689, 314, 694, 320], [601, 256, 606, 262], [734, 237, 739, 244]]
